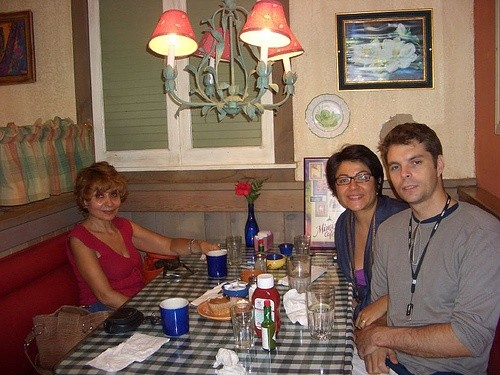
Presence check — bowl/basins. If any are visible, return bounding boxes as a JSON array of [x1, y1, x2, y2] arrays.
[[265, 253, 287, 270], [239, 269, 266, 284], [222, 279, 249, 298]]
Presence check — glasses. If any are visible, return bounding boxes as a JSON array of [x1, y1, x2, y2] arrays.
[[333, 173, 376, 186]]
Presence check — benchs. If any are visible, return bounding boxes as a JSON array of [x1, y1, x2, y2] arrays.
[[0, 228, 81, 375]]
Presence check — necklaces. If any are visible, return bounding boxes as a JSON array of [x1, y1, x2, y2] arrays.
[[406, 192, 451, 320]]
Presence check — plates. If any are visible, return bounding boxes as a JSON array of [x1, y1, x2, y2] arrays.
[[305, 93, 351, 139], [197, 296, 251, 321]]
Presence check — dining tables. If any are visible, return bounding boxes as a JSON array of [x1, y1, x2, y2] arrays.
[[52, 243, 360, 375]]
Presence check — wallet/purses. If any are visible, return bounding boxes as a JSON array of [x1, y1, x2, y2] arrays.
[[103, 308, 144, 334]]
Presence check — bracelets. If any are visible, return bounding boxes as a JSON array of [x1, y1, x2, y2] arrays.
[[189, 238, 195, 255]]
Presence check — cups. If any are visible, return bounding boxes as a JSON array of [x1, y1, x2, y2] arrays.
[[279, 243, 294, 256], [266, 253, 283, 260], [287, 255, 311, 293], [293, 235, 311, 256], [225, 235, 243, 265], [159, 297, 189, 336], [205, 248, 230, 279], [231, 302, 255, 350], [306, 283, 335, 341]]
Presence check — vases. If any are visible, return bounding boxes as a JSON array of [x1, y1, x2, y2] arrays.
[[244, 203, 259, 247]]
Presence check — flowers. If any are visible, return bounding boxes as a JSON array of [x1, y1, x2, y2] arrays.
[[233, 174, 272, 205]]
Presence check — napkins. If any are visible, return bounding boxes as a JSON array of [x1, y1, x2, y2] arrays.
[[105, 332, 170, 363], [282, 288, 321, 326], [214, 362, 248, 375], [213, 348, 240, 368], [278, 263, 327, 286], [85, 344, 136, 373], [191, 281, 227, 306]]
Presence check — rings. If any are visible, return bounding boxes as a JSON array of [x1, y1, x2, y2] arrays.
[[361, 321, 367, 323]]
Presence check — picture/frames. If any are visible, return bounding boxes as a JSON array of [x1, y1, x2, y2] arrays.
[[334, 8, 435, 92], [0, 9, 36, 86], [303, 157, 347, 250]]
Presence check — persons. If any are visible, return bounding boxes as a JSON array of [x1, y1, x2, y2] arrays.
[[65, 162, 221, 314], [325, 144, 410, 329], [352, 123, 500, 375]]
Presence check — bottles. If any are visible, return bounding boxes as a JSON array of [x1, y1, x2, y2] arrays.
[[254, 240, 266, 274], [251, 273, 280, 338], [261, 300, 276, 350], [248, 274, 258, 303]]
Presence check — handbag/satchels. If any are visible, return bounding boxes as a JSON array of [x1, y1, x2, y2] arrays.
[[23, 306, 116, 375]]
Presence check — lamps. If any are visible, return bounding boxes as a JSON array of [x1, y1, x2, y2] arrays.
[[147, 0, 306, 123]]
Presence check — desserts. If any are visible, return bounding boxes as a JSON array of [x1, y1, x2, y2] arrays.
[[208, 298, 231, 315]]
